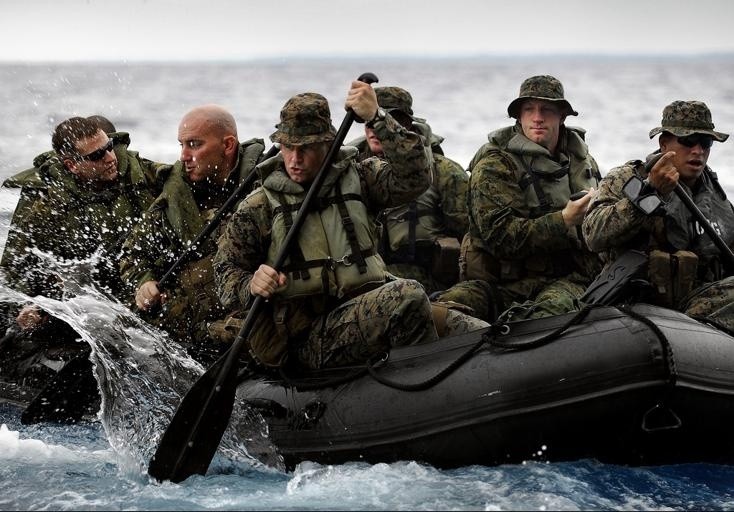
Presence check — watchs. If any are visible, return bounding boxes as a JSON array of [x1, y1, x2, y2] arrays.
[[365, 108, 387, 129]]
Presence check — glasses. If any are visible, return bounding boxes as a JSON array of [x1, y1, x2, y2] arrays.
[[76, 137, 115, 162], [679, 133, 714, 149], [621, 174, 673, 215]]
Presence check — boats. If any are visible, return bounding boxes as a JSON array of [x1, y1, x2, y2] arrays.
[[0, 285, 731, 472]]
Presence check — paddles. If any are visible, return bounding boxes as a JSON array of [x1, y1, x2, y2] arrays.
[[149, 72, 379, 483], [22, 143, 280, 424]]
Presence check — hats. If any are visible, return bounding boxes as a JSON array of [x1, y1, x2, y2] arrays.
[[372, 86, 427, 123], [411, 124, 444, 147], [508, 75, 577, 120], [649, 98, 729, 146], [270, 93, 339, 146]]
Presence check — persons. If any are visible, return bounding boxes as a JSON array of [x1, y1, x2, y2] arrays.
[[87, 115, 114, 135], [581, 98, 733, 337], [341, 86, 471, 296], [213, 78, 492, 379], [115, 102, 269, 379], [429, 73, 605, 326], [0, 117, 170, 329]]
